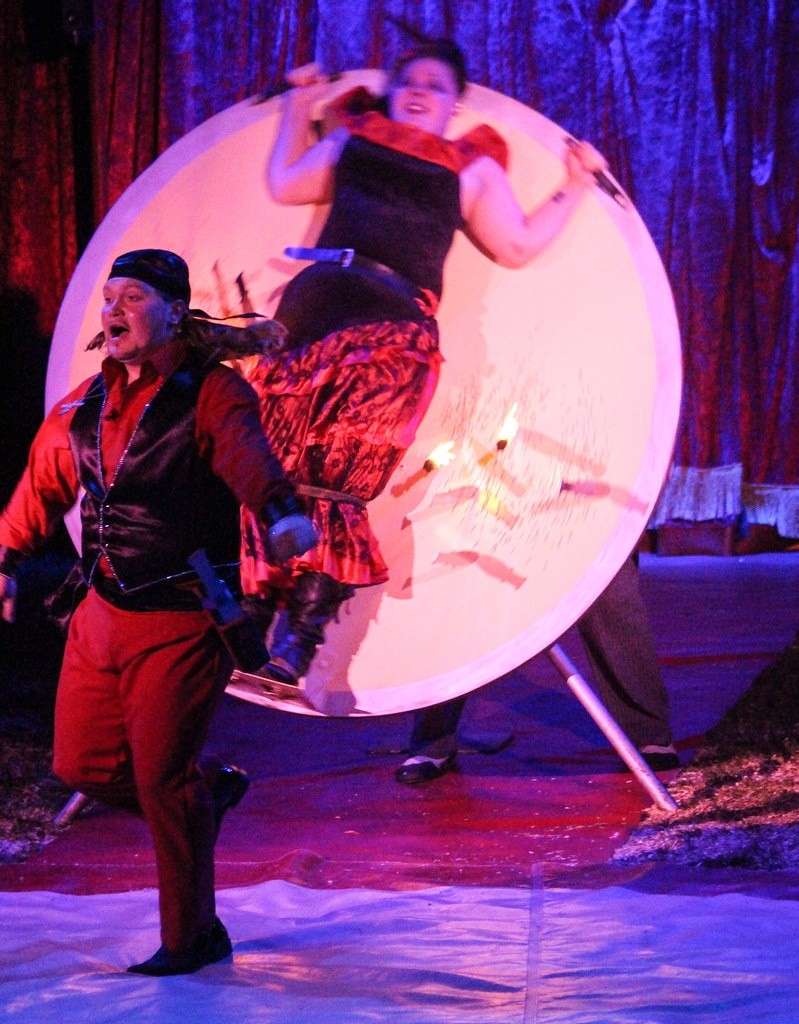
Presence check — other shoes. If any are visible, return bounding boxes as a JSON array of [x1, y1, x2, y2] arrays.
[[396, 752, 459, 784], [638, 742, 680, 770]]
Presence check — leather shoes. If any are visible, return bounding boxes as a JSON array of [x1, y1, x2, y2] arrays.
[[127, 928, 232, 977], [214, 765, 250, 832]]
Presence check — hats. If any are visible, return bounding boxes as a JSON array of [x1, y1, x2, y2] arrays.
[[107, 249, 192, 308]]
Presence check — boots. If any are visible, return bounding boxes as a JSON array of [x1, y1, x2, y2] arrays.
[[263, 572, 342, 682]]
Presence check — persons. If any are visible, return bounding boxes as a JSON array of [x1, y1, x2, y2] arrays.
[[389, 550, 683, 782], [0, 249, 324, 976], [238, 39, 611, 683]]
